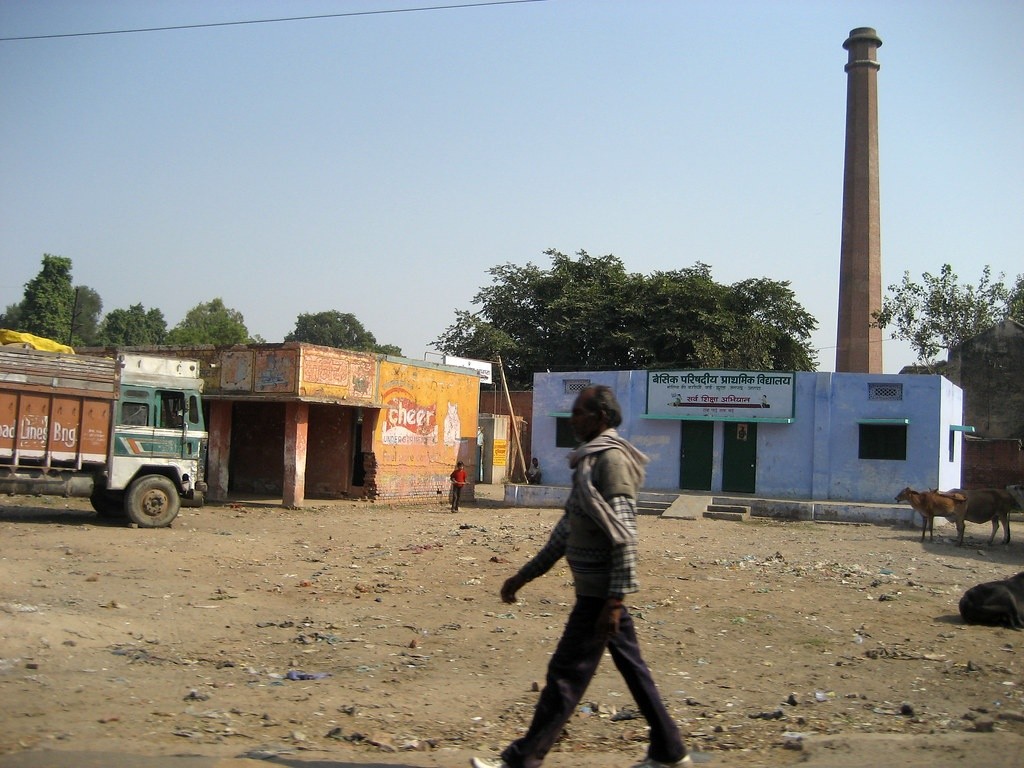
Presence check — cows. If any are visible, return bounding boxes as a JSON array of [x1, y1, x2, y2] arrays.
[[895, 486, 1022, 547]]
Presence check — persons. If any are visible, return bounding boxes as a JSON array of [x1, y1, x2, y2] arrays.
[[448, 460, 470, 514], [524, 458, 543, 484], [468, 385, 697, 768]]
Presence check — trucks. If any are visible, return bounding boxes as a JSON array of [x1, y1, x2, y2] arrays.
[[0, 340, 209, 528]]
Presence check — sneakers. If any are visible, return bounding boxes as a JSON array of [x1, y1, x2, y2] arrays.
[[631, 754, 694, 768], [470, 755, 511, 768]]
